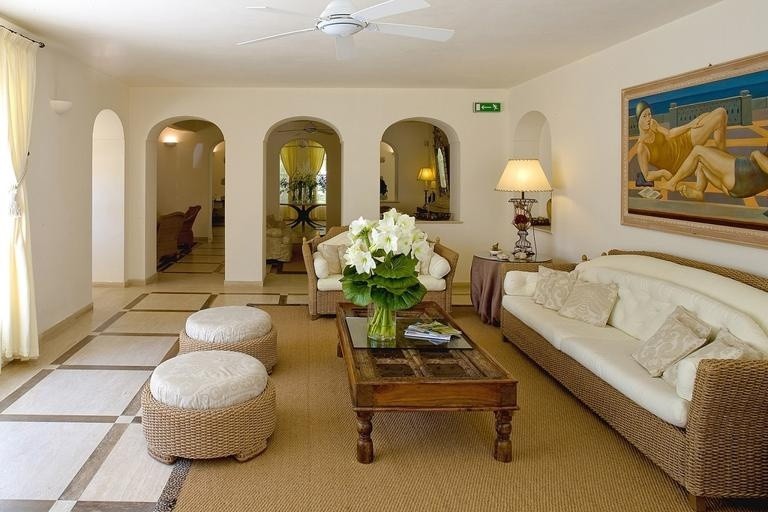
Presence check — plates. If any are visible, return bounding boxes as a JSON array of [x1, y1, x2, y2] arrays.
[[490, 250, 502, 255]]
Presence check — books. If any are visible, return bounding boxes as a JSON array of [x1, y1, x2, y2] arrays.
[[404, 319, 463, 345], [638, 186, 663, 200]]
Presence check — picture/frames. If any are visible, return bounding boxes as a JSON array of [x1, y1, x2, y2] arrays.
[[621, 51, 768, 251]]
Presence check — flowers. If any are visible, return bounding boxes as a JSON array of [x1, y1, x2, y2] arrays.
[[339, 207, 433, 308]]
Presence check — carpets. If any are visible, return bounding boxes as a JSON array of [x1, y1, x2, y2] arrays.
[[156, 304, 767, 510]]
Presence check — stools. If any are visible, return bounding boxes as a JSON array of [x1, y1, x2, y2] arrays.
[[178, 304, 279, 379], [140, 348, 277, 466]]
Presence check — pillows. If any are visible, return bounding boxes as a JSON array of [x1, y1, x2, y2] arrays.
[[421, 241, 436, 275], [556, 278, 619, 328], [664, 328, 768, 402], [532, 264, 579, 311], [322, 242, 343, 275], [631, 304, 713, 377]]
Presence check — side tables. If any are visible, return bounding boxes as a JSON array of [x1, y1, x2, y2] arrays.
[[469, 250, 552, 326]]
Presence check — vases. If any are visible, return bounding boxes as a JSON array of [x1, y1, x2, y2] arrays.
[[366, 302, 397, 342]]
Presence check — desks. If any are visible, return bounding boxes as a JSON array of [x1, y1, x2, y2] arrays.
[[280, 204, 325, 229]]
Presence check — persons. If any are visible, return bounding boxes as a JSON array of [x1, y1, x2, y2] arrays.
[[636, 99, 727, 182], [654, 141, 768, 201]]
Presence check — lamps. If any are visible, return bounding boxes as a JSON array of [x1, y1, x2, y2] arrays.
[[495, 157, 554, 259], [417, 167, 437, 209]]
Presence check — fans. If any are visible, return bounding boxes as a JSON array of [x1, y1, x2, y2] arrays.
[[233, 0, 455, 47], [278, 120, 334, 136]]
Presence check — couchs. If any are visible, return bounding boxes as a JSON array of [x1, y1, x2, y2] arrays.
[[500, 250, 768, 512], [302, 225, 460, 320]]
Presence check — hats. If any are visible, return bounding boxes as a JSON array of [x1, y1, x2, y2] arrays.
[[636, 99, 652, 120]]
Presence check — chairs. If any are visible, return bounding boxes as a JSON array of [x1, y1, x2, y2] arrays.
[[157, 211, 185, 264], [177, 205, 201, 249]]
